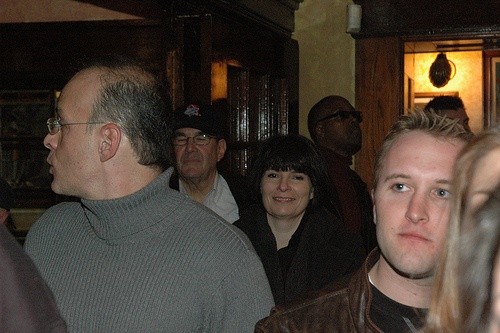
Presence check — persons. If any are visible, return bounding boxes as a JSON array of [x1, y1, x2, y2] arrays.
[[0, 176, 67, 333], [419, 124, 500, 333], [173, 102, 260, 229], [308, 95, 376, 287], [253, 107, 472, 333], [232, 133, 359, 306], [23, 56, 274, 333], [425, 95, 471, 133]]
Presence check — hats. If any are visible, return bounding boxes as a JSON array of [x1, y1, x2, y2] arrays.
[[172, 102, 224, 137]]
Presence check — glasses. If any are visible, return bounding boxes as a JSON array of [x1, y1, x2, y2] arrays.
[[47, 117, 106, 136], [174, 133, 218, 146], [316, 110, 363, 123]]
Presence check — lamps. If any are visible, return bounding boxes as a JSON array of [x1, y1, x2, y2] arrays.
[[430, 51, 457, 86]]
